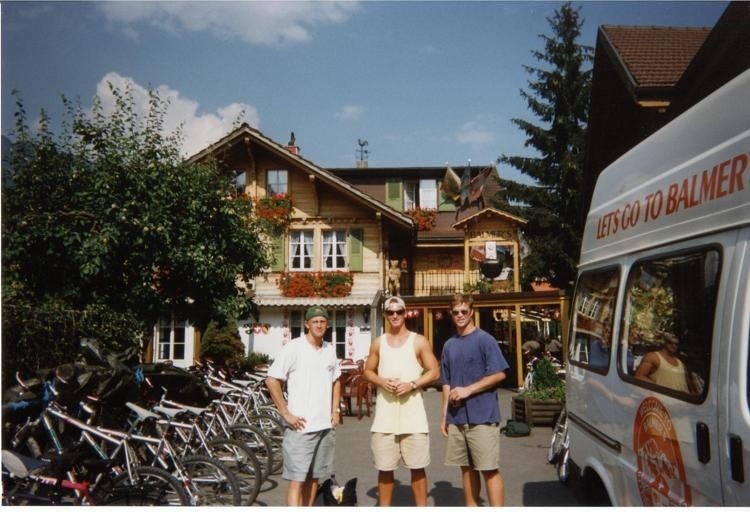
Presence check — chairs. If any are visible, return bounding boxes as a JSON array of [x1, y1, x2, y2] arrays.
[[338, 359, 378, 424]]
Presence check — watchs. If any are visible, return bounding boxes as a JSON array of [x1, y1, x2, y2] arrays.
[[408, 379, 416, 390]]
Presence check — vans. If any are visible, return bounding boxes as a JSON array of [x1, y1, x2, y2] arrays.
[[566, 68, 750, 507]]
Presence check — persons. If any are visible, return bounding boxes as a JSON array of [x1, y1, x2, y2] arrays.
[[361, 295, 440, 506], [440, 293, 511, 506], [590, 317, 632, 372], [389, 259, 402, 295], [512, 323, 564, 367], [635, 330, 698, 395], [264, 304, 342, 505]]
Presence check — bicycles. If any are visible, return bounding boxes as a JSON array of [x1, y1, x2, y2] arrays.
[[1, 361, 287, 506]]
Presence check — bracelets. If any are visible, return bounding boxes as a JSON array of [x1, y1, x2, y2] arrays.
[[332, 408, 341, 412]]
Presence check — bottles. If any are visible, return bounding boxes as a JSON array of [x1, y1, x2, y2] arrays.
[[330, 474, 338, 496]]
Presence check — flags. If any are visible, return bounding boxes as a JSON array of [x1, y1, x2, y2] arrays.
[[440, 165, 491, 207]]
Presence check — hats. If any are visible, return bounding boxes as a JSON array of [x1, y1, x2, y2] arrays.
[[382, 296, 409, 318], [305, 308, 326, 321]]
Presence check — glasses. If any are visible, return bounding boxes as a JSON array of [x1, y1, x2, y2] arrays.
[[386, 308, 405, 317], [666, 338, 679, 346], [450, 308, 469, 317]]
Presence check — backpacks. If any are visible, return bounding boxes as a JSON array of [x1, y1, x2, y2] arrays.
[[319, 473, 358, 507]]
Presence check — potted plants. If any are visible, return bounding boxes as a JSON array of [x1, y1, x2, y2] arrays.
[[512, 352, 566, 427]]
